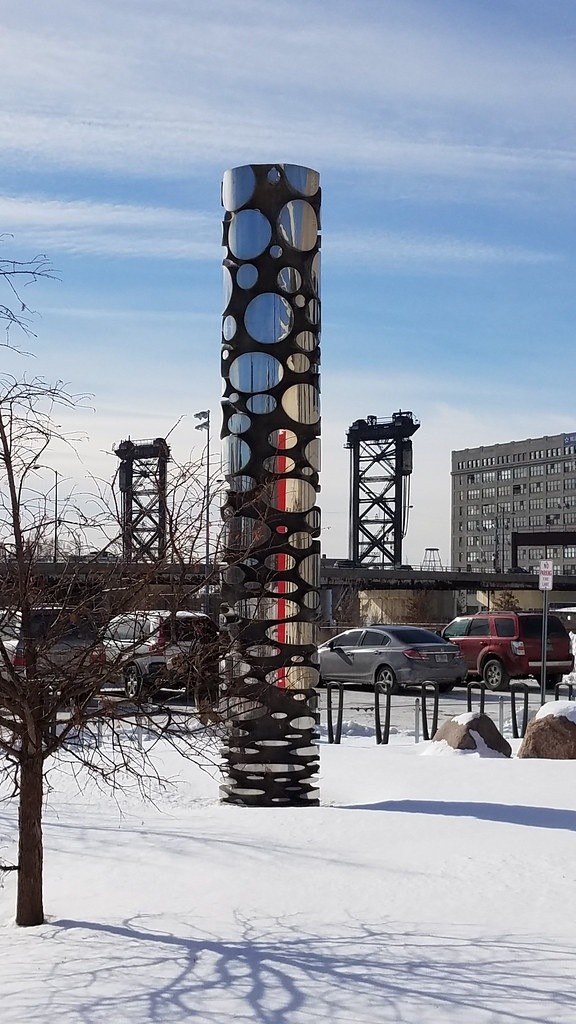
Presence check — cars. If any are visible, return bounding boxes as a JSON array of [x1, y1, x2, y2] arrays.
[[317, 625, 467, 697]]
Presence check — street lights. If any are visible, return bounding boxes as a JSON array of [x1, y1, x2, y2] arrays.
[[196, 410, 212, 603]]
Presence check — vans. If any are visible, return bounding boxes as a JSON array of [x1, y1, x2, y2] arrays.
[[0, 601, 107, 712]]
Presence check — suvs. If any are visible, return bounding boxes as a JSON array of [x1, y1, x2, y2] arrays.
[[97, 609, 222, 700], [434, 612, 575, 692]]
